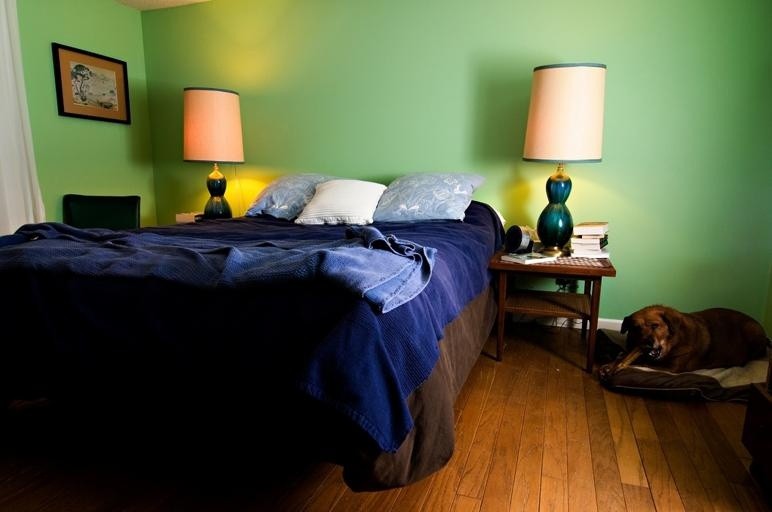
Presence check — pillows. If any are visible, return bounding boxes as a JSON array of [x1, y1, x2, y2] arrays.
[[246, 172, 488, 227]]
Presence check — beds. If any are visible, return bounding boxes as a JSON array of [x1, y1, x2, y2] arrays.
[[1, 199, 505, 491]]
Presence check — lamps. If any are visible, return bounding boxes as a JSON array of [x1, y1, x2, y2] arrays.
[[182, 85, 244, 218], [521, 63, 607, 255]]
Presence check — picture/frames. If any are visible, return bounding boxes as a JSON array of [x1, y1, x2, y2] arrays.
[[51, 42, 132, 124]]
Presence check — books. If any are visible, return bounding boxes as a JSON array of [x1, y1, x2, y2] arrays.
[[500, 251, 557, 265], [568, 221, 611, 259]]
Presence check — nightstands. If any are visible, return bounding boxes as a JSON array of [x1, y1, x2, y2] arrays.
[[488, 245, 617, 373]]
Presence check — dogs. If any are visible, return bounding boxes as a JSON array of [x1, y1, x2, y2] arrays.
[[596, 304, 772, 383]]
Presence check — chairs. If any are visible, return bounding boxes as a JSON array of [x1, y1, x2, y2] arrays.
[[60, 193, 141, 231]]
